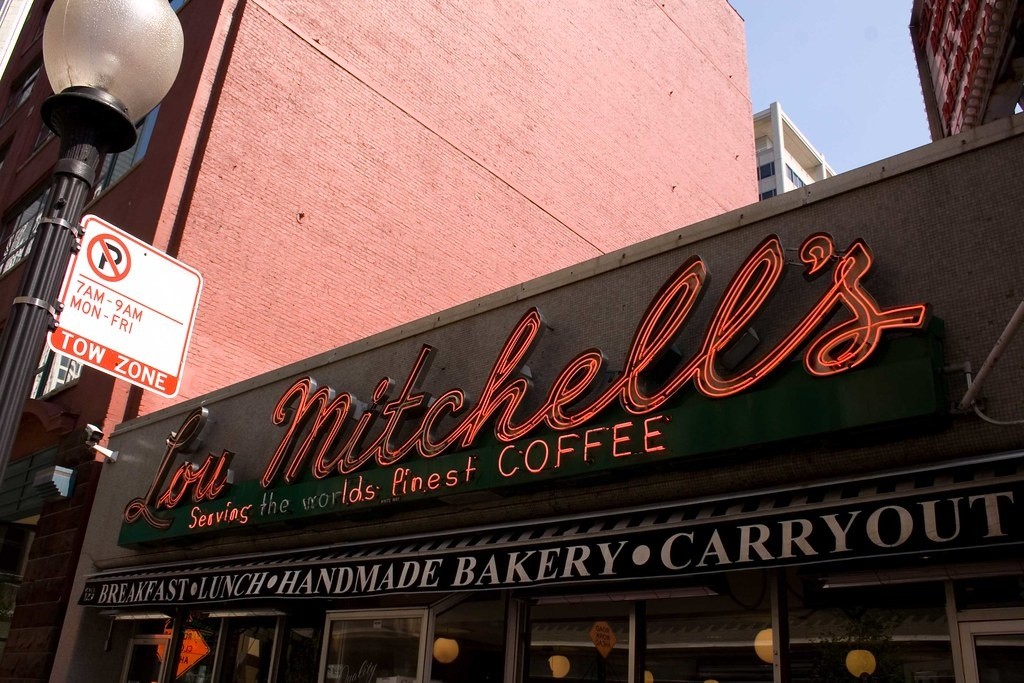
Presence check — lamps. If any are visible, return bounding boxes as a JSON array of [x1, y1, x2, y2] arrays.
[[548, 646, 570, 678], [432, 627, 459, 664]]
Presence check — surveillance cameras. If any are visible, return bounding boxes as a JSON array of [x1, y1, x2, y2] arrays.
[[85, 424, 104, 440]]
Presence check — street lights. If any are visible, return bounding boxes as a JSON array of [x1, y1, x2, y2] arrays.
[[0, 0, 186, 482]]
